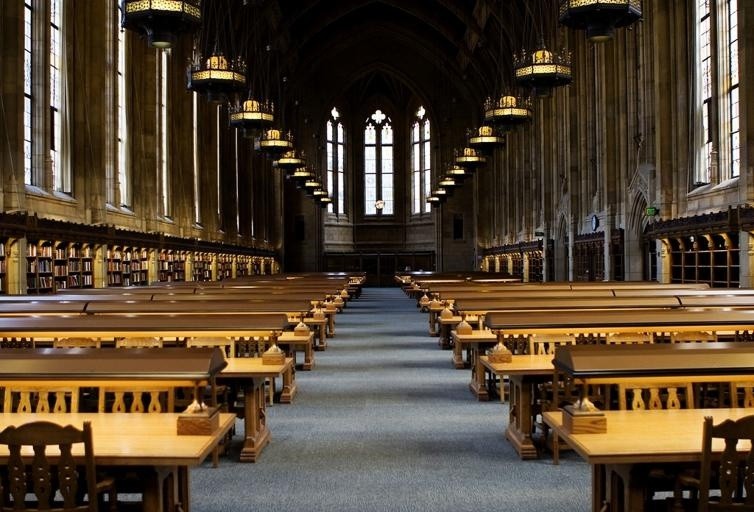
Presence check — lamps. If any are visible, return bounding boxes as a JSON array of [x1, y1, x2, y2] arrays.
[[123, 1, 332, 207], [426, 2, 642, 204]]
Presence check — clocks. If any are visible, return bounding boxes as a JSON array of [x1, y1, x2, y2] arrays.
[[591, 215, 597, 231]]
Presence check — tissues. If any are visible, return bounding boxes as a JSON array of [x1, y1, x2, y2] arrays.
[[176, 288, 347, 435], [410, 281, 608, 435]]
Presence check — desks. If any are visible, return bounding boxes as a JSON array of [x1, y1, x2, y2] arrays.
[[396, 271, 753, 512], [0, 272, 365, 512]]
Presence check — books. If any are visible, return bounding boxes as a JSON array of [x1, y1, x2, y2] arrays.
[[1, 237, 274, 296]]
